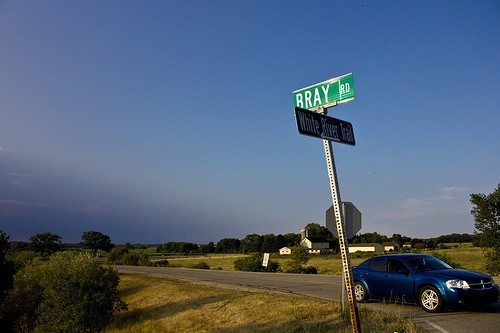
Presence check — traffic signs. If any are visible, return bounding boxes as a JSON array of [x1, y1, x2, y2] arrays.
[[294, 106, 357, 147], [291, 71, 356, 112]]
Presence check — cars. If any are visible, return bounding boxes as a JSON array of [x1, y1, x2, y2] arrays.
[[345, 253, 499, 314]]
